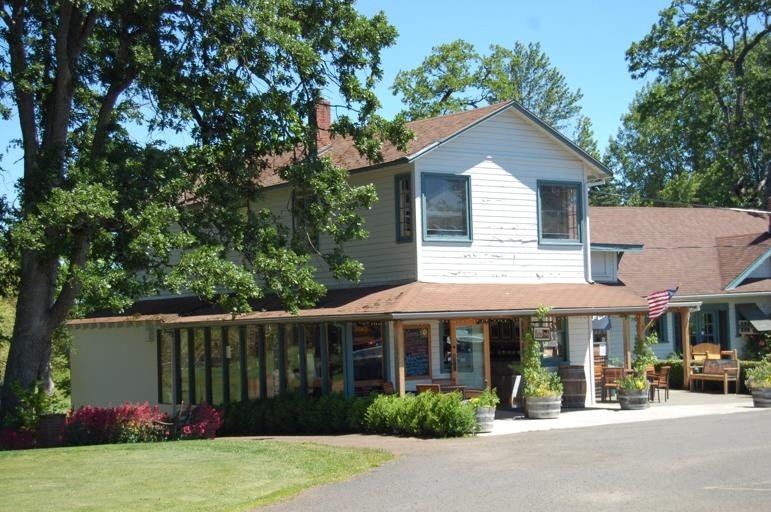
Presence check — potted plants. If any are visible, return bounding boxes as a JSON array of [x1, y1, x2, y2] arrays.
[[618, 374, 650, 410], [749, 365, 771, 408], [523, 367, 563, 419], [471, 387, 501, 434]]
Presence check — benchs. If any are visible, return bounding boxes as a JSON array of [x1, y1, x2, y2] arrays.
[[688, 342, 740, 394]]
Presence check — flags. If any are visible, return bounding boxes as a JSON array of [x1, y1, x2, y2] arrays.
[[645, 289, 678, 320]]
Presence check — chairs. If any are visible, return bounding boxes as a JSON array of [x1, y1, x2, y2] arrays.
[[602, 366, 671, 404], [380, 377, 482, 401]]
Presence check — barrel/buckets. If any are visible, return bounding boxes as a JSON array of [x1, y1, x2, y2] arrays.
[[473, 404, 497, 434], [526, 392, 564, 418], [616, 385, 654, 410], [558, 364, 588, 408], [749, 386, 771, 408]]
